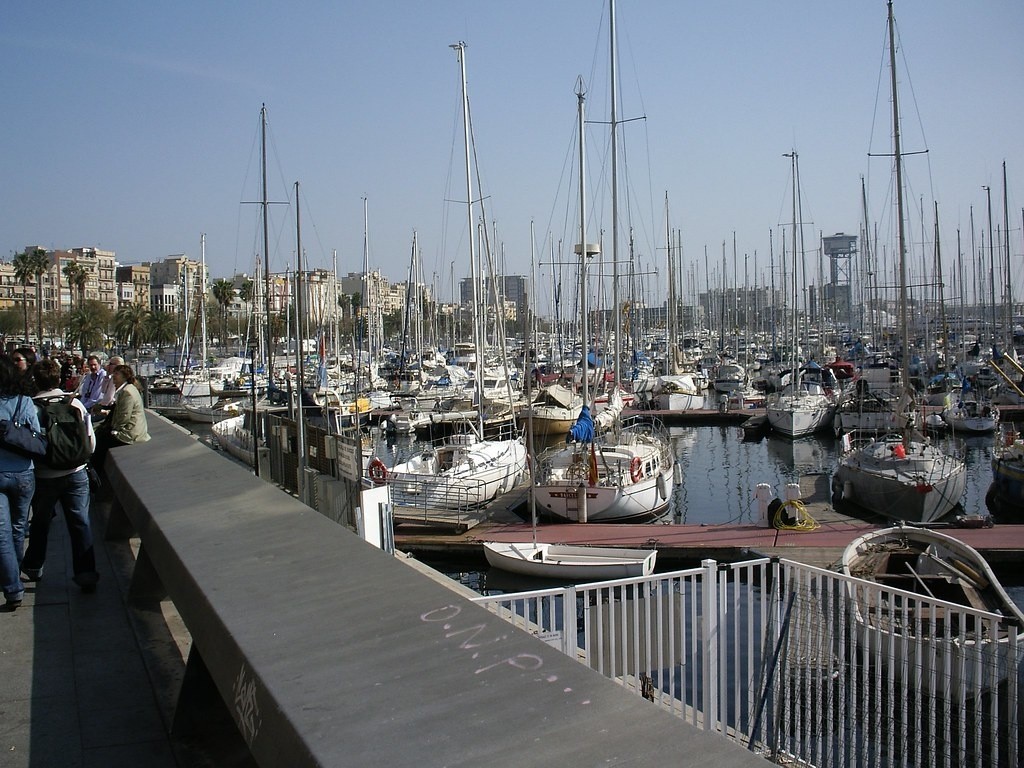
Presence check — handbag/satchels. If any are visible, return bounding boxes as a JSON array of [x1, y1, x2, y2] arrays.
[[0, 395, 46, 460], [89, 468, 102, 493]]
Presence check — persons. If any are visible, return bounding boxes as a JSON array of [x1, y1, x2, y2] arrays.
[[0, 346, 152, 613]]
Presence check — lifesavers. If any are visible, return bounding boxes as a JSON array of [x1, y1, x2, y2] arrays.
[[630, 457, 645, 482], [368, 461, 389, 483]]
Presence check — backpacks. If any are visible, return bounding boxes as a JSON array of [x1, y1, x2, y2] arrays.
[[32, 394, 91, 471]]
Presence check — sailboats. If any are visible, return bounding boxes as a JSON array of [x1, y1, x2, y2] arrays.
[[0, 0, 1024, 528]]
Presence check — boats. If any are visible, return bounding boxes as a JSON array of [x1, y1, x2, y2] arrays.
[[481, 539, 659, 582], [840, 520, 1023, 701]]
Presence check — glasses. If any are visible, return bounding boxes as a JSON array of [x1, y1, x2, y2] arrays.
[[12, 357, 26, 362], [109, 362, 115, 365]]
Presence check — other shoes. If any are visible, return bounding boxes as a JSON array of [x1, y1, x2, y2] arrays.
[[20, 560, 44, 582], [6, 599, 21, 606], [81, 587, 97, 592]]
